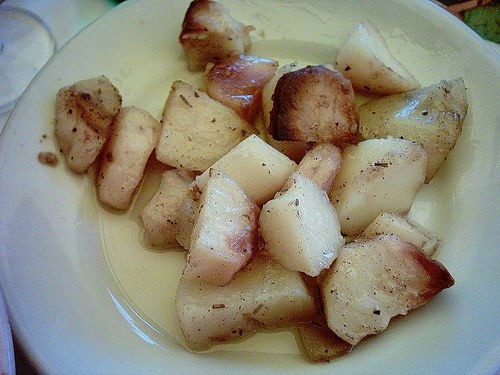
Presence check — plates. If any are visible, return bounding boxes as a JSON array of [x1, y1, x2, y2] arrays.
[[0, 0, 500, 375]]
